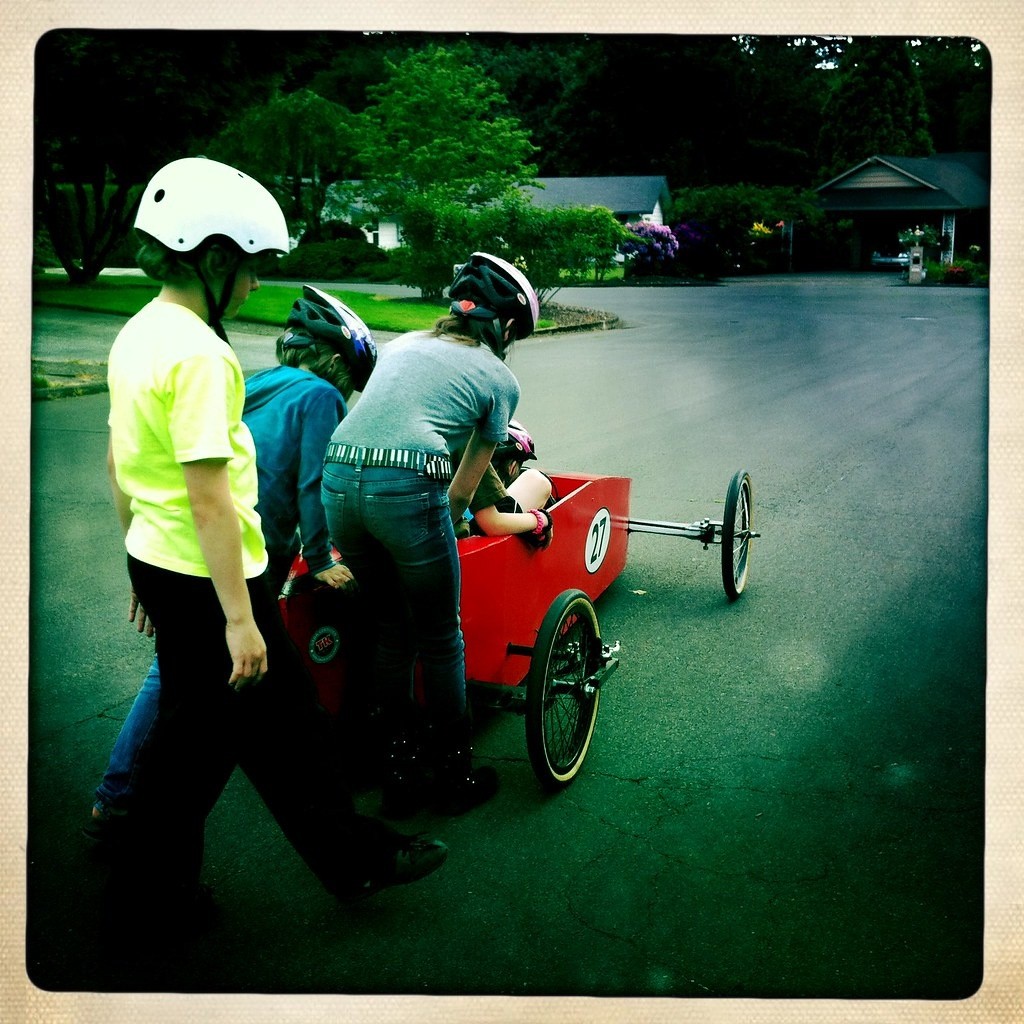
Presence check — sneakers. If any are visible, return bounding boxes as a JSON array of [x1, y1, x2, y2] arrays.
[[325, 837, 452, 901], [81, 794, 141, 839]]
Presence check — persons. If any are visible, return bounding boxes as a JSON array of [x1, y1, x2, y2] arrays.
[[86, 153, 451, 938], [74, 285, 377, 826], [320, 251, 541, 823], [449, 426, 553, 555], [489, 425, 557, 511]]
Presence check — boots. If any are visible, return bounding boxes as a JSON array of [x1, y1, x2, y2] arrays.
[[363, 690, 448, 825], [421, 697, 501, 817]]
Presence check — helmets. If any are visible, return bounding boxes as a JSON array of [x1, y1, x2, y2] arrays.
[[491, 423, 538, 465], [283, 282, 377, 393], [133, 152, 294, 263], [445, 251, 540, 359]]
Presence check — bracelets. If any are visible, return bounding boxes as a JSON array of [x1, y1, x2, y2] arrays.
[[537, 509, 553, 532], [527, 510, 543, 535]]
